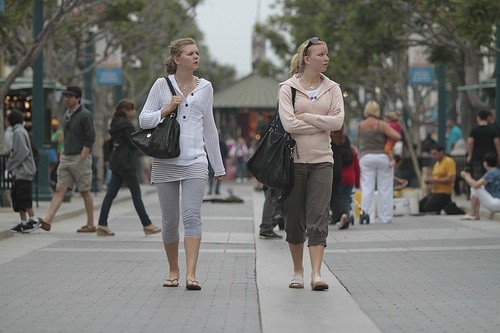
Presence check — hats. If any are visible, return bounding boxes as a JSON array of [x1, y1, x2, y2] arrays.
[[62, 86, 82, 97]]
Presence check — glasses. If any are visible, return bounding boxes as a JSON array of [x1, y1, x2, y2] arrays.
[[304, 37, 321, 55]]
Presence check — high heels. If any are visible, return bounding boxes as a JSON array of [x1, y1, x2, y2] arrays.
[[97, 227, 115, 237], [143, 228, 161, 235]]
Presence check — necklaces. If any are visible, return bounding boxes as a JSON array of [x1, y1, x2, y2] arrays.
[[300, 76, 321, 90]]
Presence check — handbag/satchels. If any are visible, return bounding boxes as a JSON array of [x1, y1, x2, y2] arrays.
[[102, 138, 112, 161], [129, 76, 180, 159], [247, 87, 296, 192]]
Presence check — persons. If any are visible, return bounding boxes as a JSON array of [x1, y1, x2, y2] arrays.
[[104, 129, 114, 187], [208, 128, 269, 197], [49, 118, 65, 187], [329, 98, 500, 230], [134, 35, 229, 294], [4, 107, 42, 235], [275, 35, 345, 293], [96, 96, 161, 238], [34, 84, 97, 233], [0, 115, 15, 190], [177, 79, 196, 93], [258, 188, 283, 241]]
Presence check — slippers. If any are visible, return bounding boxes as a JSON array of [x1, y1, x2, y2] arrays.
[[163, 278, 178, 287], [186, 279, 201, 290]]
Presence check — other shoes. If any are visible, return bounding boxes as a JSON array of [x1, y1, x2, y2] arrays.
[[359, 214, 370, 225], [310, 281, 329, 291], [258, 232, 282, 239], [39, 218, 50, 231], [22, 219, 42, 233], [77, 225, 95, 232], [289, 279, 304, 289], [460, 214, 480, 221], [339, 215, 354, 229], [8, 222, 26, 233]]
